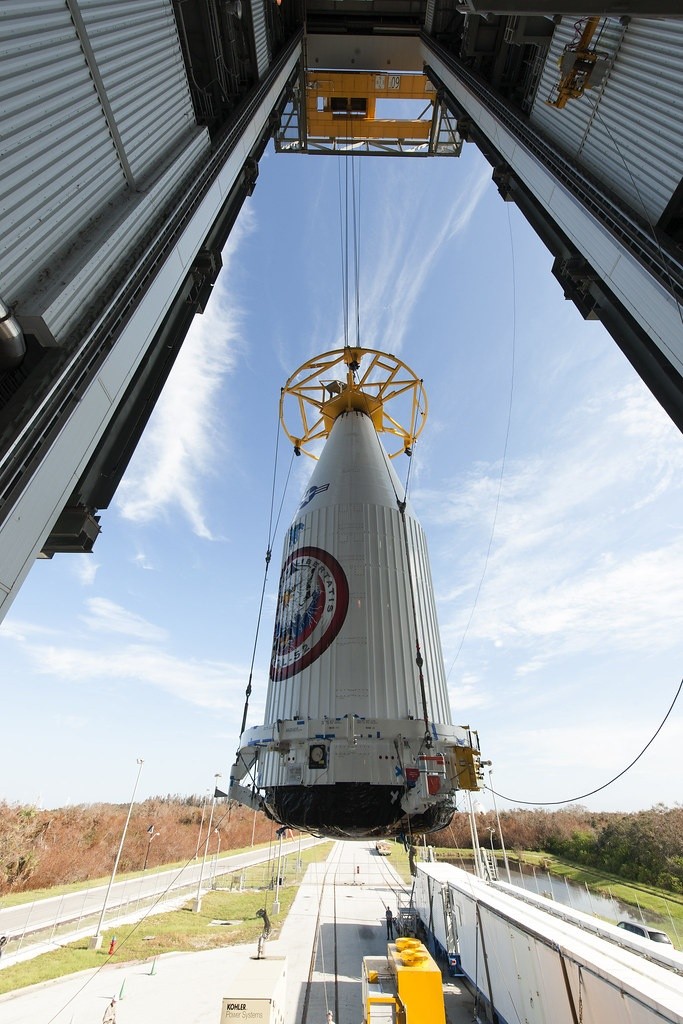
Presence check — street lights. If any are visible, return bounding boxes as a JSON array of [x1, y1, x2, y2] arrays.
[[489, 769, 511, 883], [190, 773, 222, 912], [86, 756, 146, 952]]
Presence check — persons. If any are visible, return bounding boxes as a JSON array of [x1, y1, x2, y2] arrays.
[[386, 906, 395, 941]]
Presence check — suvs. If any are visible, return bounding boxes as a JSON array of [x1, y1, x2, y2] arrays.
[[616, 921, 673, 948]]
